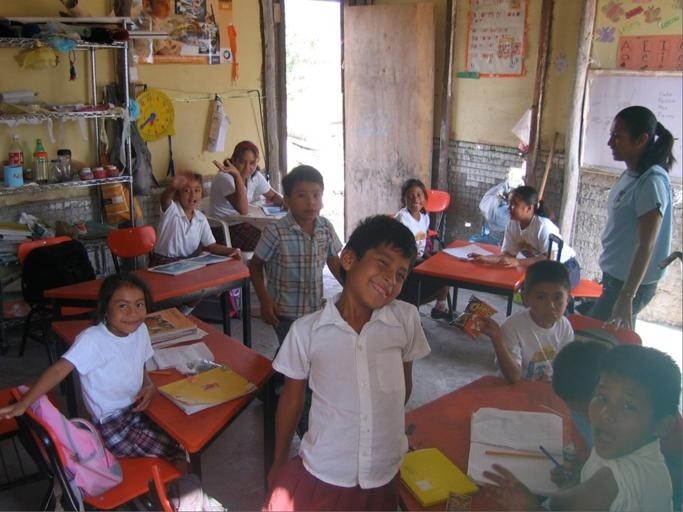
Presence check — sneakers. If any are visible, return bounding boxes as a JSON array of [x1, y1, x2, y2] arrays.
[[430, 307, 450, 319]]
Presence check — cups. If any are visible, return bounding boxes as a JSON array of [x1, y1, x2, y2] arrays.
[[4, 165, 24, 187]]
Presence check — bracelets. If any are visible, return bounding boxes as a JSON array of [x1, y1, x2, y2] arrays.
[[620, 288, 636, 300], [616, 288, 637, 300]]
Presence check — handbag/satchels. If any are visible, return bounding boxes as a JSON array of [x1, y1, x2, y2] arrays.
[[101, 183, 143, 224], [20, 239, 95, 295]]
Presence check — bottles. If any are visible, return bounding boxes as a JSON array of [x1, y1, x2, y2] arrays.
[[9, 133, 119, 182]]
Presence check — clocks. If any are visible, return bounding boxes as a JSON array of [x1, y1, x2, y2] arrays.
[[133, 90, 176, 141]]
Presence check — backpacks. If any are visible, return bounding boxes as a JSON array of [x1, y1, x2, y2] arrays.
[[573, 328, 618, 350], [165, 473, 226, 512], [17, 383, 121, 497]]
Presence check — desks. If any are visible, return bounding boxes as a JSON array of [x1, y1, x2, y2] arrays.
[[43, 252, 252, 348], [49, 310, 283, 496], [395, 374, 590, 512], [412, 239, 529, 318]]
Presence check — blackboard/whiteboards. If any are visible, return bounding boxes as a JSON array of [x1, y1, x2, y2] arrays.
[[579, 69, 683, 184]]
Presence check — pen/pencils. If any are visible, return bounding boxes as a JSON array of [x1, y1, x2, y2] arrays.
[[541, 405, 565, 417], [540, 446, 561, 467], [486, 451, 547, 458], [152, 371, 171, 375]]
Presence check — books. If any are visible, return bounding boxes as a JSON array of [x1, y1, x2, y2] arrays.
[[155, 366, 259, 415], [140, 308, 206, 349], [147, 252, 235, 277], [398, 448, 479, 506], [467, 408, 563, 494]]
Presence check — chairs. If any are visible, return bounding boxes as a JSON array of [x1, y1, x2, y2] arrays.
[[16, 235, 94, 366], [564, 311, 642, 352], [106, 226, 156, 273], [385, 212, 453, 319], [566, 277, 608, 311], [196, 196, 287, 250], [1, 298, 32, 356], [9, 386, 186, 512], [0, 383, 65, 490], [422, 187, 453, 248]]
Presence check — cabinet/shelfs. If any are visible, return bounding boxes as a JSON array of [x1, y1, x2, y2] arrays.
[[0, 17, 137, 285]]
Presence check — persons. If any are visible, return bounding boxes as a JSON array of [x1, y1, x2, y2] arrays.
[[249, 165, 345, 402], [265, 216, 432, 512], [209, 142, 289, 324], [0, 274, 227, 511], [394, 179, 458, 319], [481, 260, 574, 385], [588, 106, 676, 335], [148, 171, 243, 316], [466, 186, 585, 293], [551, 339, 683, 498], [482, 344, 681, 511]]
